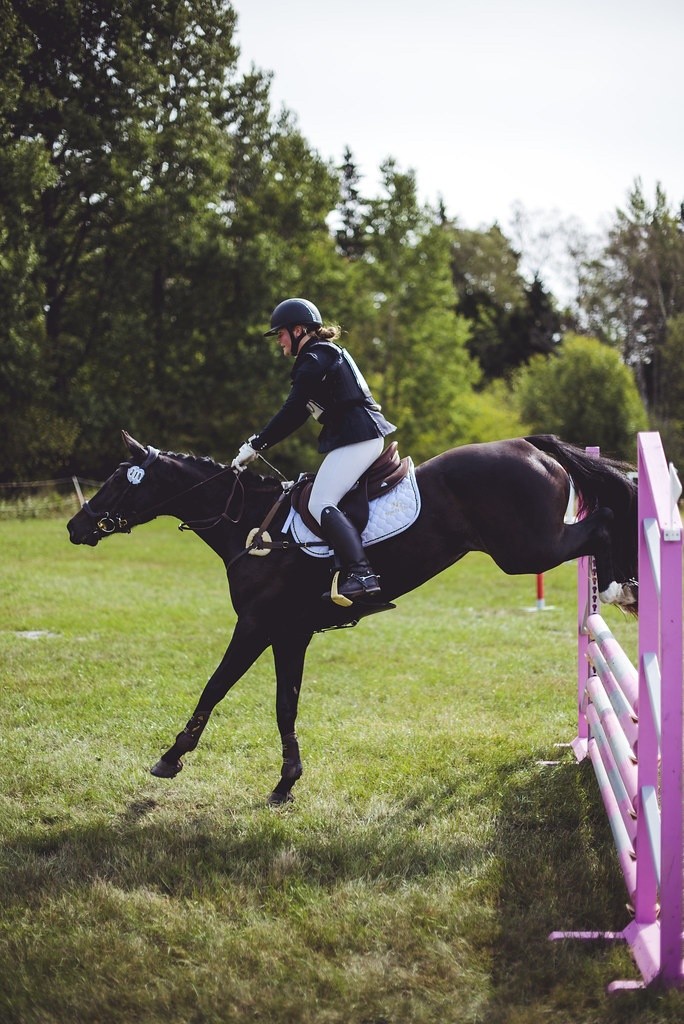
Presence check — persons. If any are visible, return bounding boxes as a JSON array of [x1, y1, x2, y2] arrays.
[[231, 297, 386, 602]]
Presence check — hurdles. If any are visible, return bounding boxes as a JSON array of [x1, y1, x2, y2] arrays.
[[542, 428, 682, 999]]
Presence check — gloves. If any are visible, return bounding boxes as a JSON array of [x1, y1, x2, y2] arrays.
[[231, 443, 258, 474]]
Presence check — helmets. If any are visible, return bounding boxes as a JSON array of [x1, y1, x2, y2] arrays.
[[263, 298, 323, 337]]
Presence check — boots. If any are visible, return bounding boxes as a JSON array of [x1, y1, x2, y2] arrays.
[[320, 506, 381, 601]]
